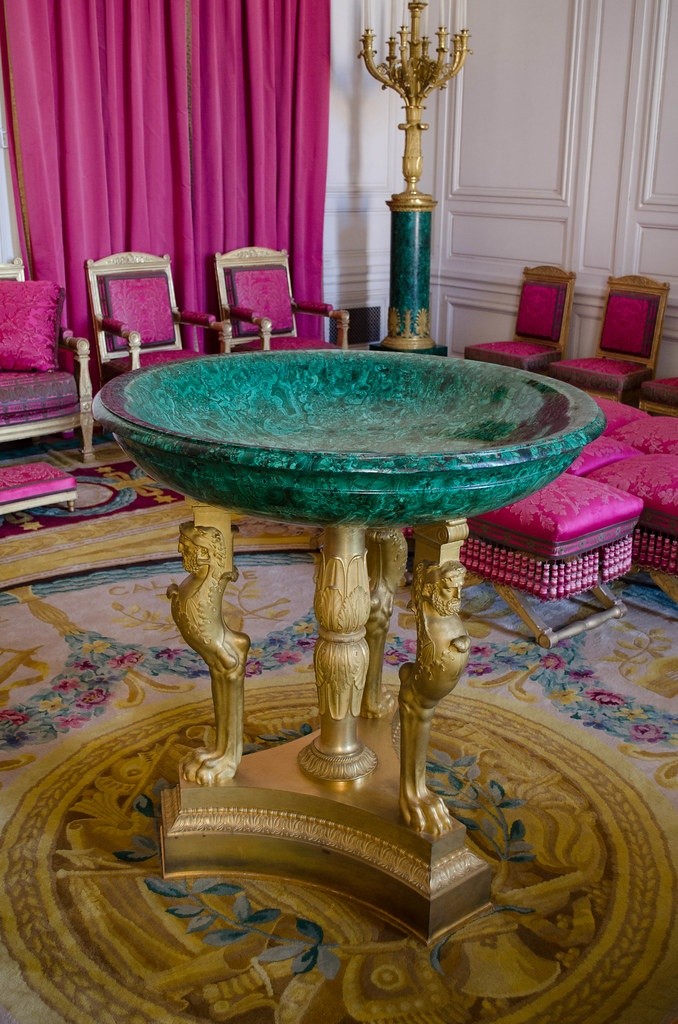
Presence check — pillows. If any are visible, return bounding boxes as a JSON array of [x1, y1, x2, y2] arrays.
[[0, 280, 65, 371]]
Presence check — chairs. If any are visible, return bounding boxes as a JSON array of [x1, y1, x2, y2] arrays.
[[637, 376, 677, 418], [548, 276, 670, 403], [214, 246, 350, 353], [84, 251, 233, 389], [463, 267, 576, 374], [0, 257, 94, 524]]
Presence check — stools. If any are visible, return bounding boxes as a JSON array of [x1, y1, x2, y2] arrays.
[[563, 392, 677, 604], [460, 473, 643, 647]]
